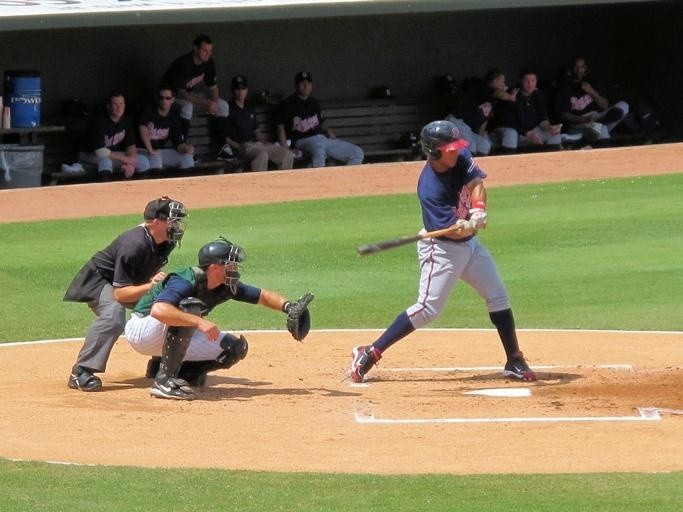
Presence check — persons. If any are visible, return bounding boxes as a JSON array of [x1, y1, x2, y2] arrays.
[[157, 35, 240, 166], [348, 117, 540, 384], [273, 70, 368, 169], [61, 193, 188, 394], [74, 90, 151, 182], [442, 55, 630, 158], [130, 81, 195, 173], [224, 74, 294, 174], [122, 231, 316, 402]]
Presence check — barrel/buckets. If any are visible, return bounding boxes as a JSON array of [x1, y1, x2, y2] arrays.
[[4, 77, 41, 127]]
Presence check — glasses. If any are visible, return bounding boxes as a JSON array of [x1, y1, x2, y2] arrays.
[[159, 95, 172, 100]]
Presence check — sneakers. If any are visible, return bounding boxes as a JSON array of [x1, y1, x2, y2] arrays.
[[591, 121, 602, 136], [150, 372, 196, 400], [215, 143, 234, 161], [146, 355, 162, 378], [503, 350, 536, 382], [350, 344, 382, 384], [68, 365, 102, 392], [193, 154, 203, 163]]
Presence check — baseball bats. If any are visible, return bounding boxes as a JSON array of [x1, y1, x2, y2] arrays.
[[356, 224, 464, 255]]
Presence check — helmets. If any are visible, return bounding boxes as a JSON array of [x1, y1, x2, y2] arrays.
[[198, 240, 248, 267], [420, 120, 471, 152]]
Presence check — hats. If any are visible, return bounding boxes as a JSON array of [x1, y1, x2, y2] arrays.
[[295, 71, 312, 84], [144, 198, 188, 219], [373, 87, 396, 99], [232, 75, 248, 89]]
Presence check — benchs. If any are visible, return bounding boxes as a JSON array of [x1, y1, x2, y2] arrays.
[[320, 101, 465, 163], [49, 105, 307, 177]]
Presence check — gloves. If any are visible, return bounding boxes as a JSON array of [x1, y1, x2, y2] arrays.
[[456, 212, 488, 236]]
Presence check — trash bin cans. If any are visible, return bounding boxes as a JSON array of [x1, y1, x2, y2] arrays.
[[0, 144, 45, 189]]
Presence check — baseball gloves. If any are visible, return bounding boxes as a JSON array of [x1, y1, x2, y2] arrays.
[[287, 292, 315, 341]]
